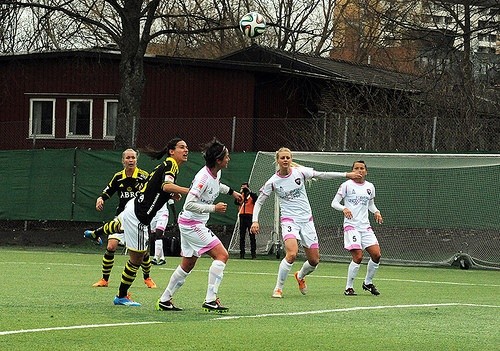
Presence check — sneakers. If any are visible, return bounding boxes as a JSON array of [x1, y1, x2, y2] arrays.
[[201, 299, 229, 313], [92, 279, 108, 287], [157, 259, 166, 265], [113, 296, 141, 306], [84, 230, 102, 245], [344, 288, 357, 295], [295, 271, 307, 294], [272, 289, 282, 298], [362, 281, 380, 295], [151, 259, 158, 265], [156, 299, 182, 311], [144, 277, 157, 288]]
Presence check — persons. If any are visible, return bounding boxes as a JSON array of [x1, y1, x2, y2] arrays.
[[156, 138, 243, 313], [233, 182, 259, 259], [91, 147, 158, 289], [83, 139, 191, 307], [249, 147, 362, 298], [331, 161, 384, 296]]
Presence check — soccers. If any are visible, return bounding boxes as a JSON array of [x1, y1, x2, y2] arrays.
[[240, 12, 266, 38]]
